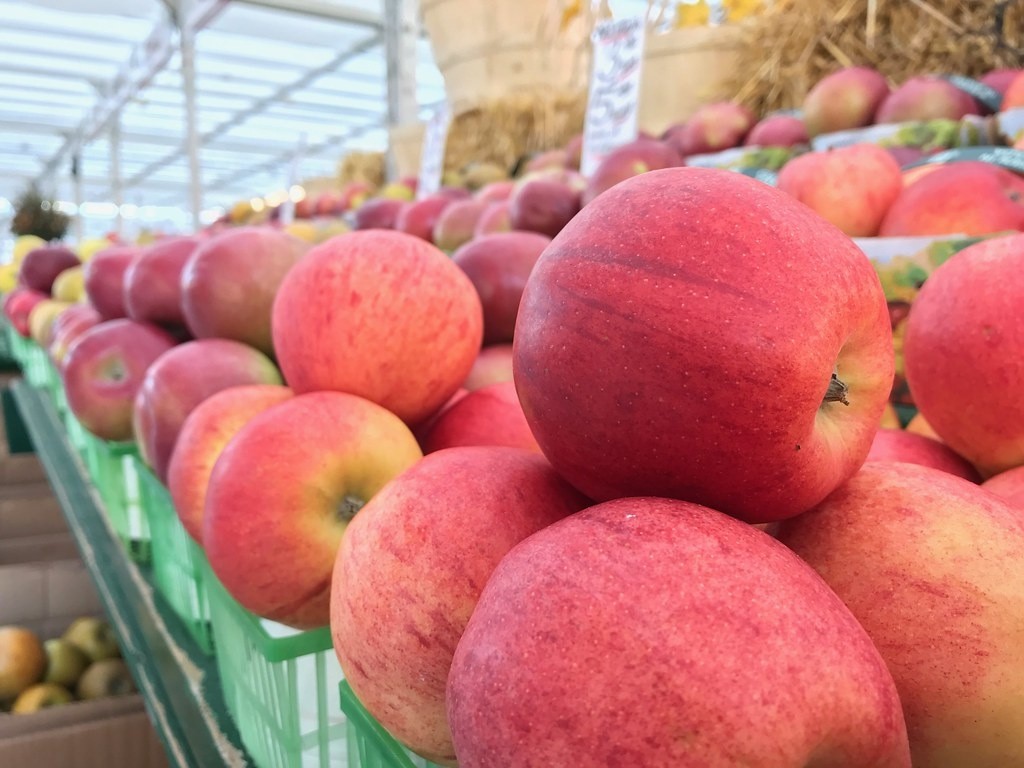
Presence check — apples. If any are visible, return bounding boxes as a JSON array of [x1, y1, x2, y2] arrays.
[[0, 66, 1024, 768]]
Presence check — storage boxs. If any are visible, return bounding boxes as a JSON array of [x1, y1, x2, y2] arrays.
[[0, 316, 443, 768]]
